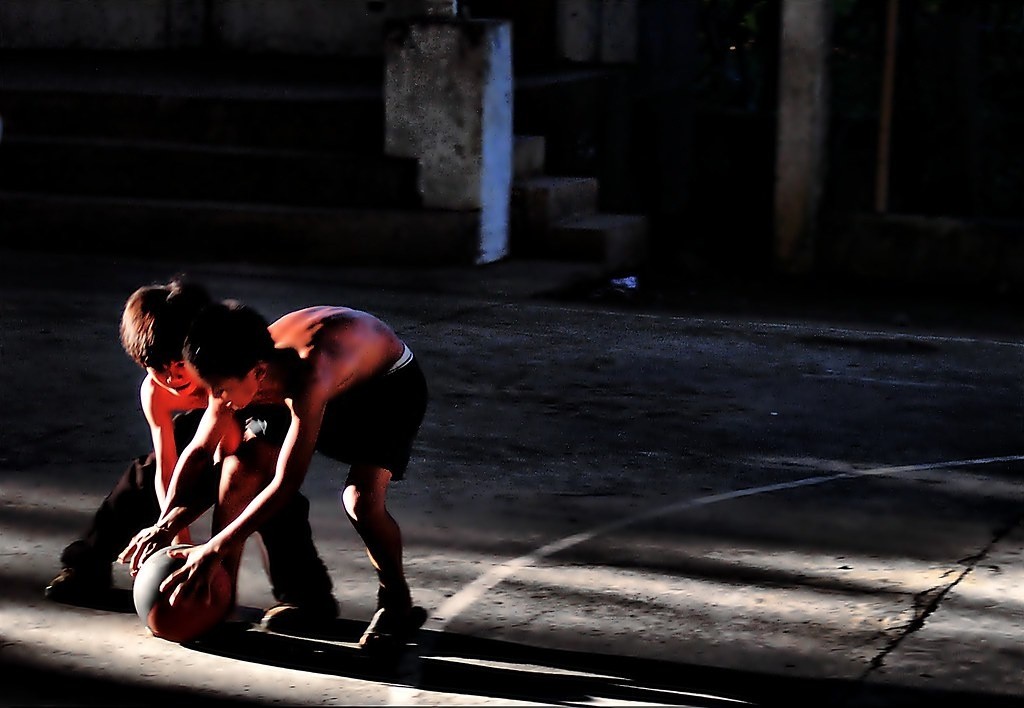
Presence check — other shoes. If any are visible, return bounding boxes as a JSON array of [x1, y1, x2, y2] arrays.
[[360, 601, 428, 646], [44, 568, 114, 599], [261, 595, 339, 632]]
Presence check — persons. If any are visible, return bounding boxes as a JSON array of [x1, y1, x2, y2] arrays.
[[118, 299, 429, 652], [43, 277, 342, 636]]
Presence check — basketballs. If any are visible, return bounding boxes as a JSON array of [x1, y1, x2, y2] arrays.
[[133, 543, 232, 640]]
